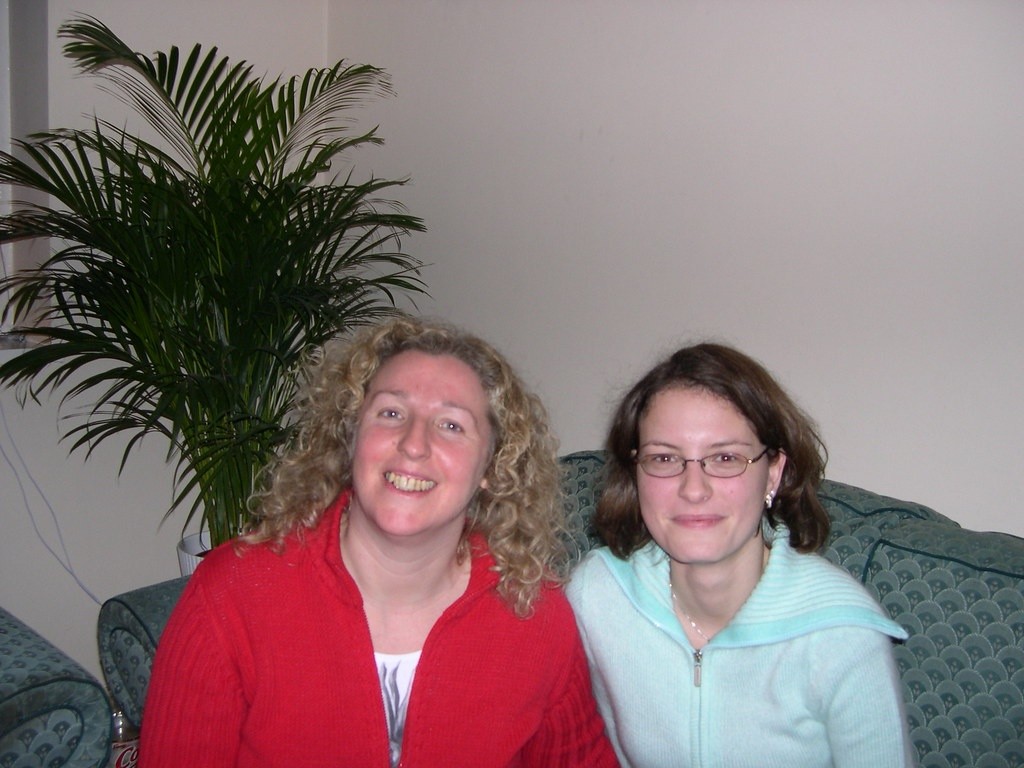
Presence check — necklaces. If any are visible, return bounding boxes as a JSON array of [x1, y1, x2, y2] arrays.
[[670, 584, 709, 641]]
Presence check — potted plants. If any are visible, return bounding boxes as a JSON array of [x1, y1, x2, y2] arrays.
[[1, 12, 428, 573]]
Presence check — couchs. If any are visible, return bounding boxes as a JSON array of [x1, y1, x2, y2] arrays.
[[0, 452, 1024, 768]]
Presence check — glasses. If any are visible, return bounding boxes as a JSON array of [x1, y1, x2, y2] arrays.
[[634, 447, 770, 479]]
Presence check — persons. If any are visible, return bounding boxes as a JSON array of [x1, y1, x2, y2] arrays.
[[561, 341, 915, 768], [139, 310, 622, 768]]
